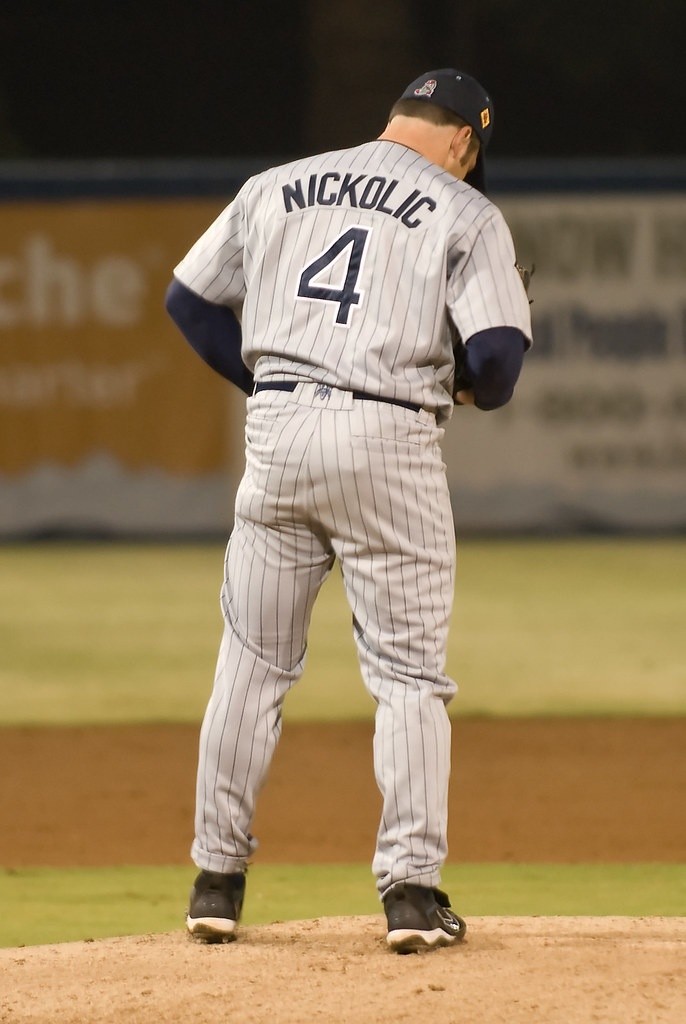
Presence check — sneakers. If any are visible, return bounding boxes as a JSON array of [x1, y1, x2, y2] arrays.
[[383, 882, 467, 947], [187, 869, 246, 942]]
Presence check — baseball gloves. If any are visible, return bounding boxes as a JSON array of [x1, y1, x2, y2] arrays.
[[515, 262, 537, 306]]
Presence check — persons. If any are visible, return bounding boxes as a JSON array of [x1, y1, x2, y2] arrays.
[[166, 69, 532, 955]]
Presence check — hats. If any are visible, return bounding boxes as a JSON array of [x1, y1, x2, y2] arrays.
[[402, 68, 494, 193]]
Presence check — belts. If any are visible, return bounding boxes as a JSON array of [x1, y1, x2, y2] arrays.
[[254, 380, 422, 412]]
